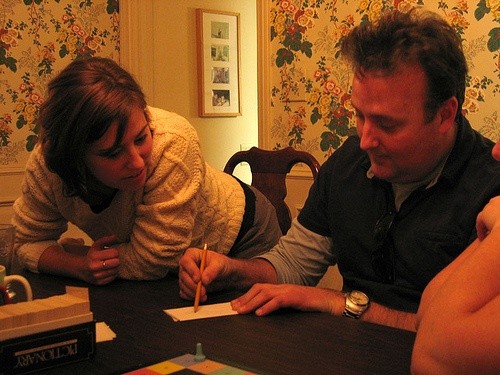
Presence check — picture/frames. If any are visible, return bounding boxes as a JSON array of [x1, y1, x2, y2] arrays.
[[196, 8, 242, 117]]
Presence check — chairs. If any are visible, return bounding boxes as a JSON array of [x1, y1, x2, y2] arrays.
[[224, 146, 320, 235]]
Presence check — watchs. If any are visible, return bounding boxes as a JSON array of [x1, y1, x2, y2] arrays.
[[342, 290, 372, 318]]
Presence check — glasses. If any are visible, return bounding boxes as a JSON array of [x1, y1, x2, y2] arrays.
[[372, 211, 396, 285]]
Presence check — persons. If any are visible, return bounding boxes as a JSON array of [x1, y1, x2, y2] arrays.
[[410, 138, 500, 375], [178, 10, 500, 334], [7, 56, 284, 289]]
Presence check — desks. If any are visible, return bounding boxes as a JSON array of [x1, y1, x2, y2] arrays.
[[8, 268, 417, 375]]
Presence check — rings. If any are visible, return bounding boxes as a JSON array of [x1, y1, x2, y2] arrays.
[[103, 261, 107, 269]]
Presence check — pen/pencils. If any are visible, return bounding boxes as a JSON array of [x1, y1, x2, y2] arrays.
[[194, 242, 207, 313]]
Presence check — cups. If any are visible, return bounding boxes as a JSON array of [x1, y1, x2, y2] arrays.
[[0, 265, 33, 301], [0, 224, 16, 273]]
